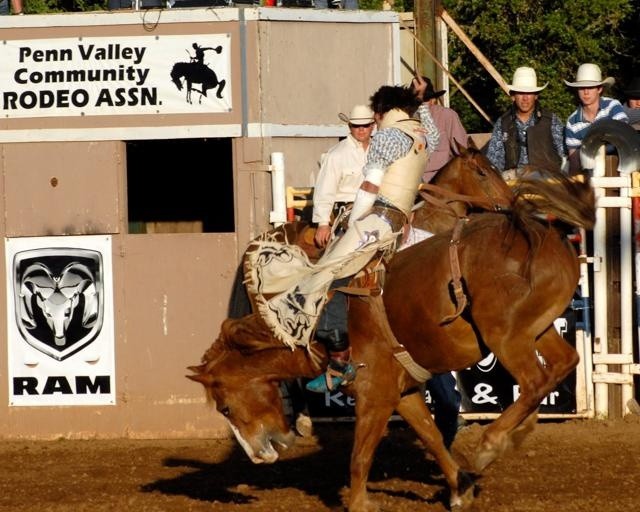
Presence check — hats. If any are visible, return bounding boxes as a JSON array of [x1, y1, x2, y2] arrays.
[[623, 78, 640, 95], [502, 65, 550, 93], [338, 104, 375, 125], [409, 76, 446, 101], [563, 63, 616, 88]]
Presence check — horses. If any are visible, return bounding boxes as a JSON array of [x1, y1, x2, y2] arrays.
[[184, 159, 596, 511], [226, 132, 540, 462]]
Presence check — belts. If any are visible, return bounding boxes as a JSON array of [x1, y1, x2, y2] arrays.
[[373, 199, 402, 211]]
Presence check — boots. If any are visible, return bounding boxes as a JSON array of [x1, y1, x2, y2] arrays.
[[305, 329, 355, 393]]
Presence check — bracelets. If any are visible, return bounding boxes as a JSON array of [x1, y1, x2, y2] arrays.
[[12, 10, 23, 16]]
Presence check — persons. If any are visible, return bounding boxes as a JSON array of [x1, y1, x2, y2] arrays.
[[0, 1, 26, 17], [408, 73, 469, 183], [243, 68, 441, 395], [309, 103, 377, 251], [621, 74, 640, 109], [560, 59, 626, 162], [488, 66, 568, 175]]
